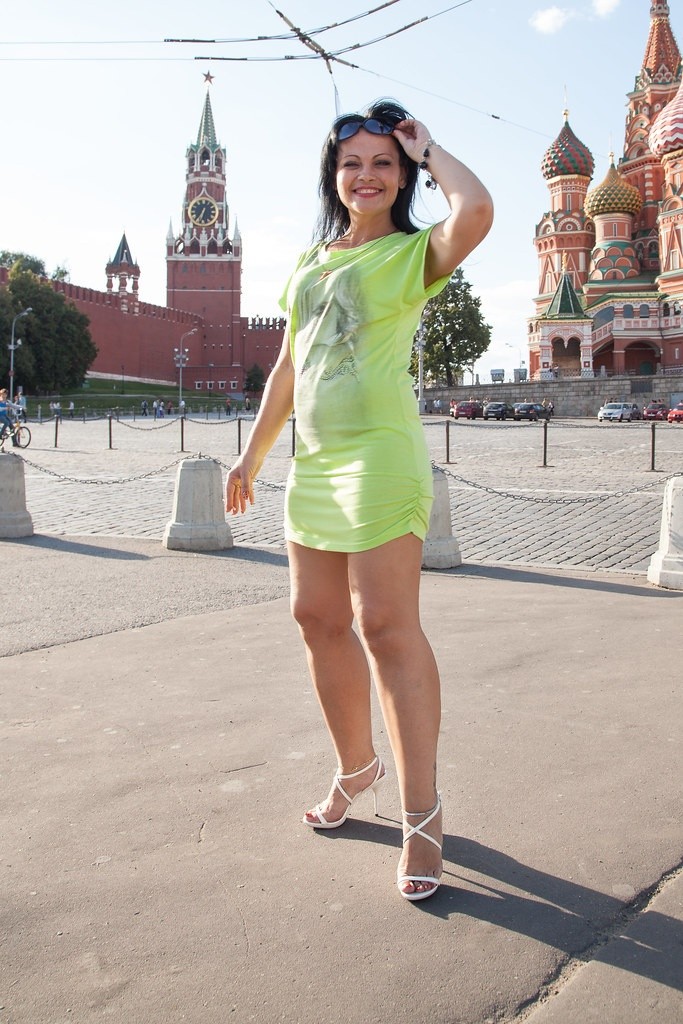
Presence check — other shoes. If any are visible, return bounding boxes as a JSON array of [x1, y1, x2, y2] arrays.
[[12, 443, 21, 447]]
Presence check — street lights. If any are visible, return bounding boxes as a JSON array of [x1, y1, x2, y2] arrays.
[[9, 307, 33, 402], [179, 328, 198, 403]]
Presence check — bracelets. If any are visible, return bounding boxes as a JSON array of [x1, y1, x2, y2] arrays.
[[420, 139, 438, 190]]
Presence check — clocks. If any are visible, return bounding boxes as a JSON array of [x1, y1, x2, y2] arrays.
[[188, 197, 219, 226]]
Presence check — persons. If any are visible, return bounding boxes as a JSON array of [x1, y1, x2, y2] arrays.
[[141, 399, 185, 418], [14, 392, 27, 423], [433, 397, 444, 415], [49, 400, 74, 418], [524, 398, 536, 404], [678, 400, 683, 405], [470, 396, 481, 404], [449, 398, 458, 416], [548, 363, 559, 380], [604, 397, 617, 405], [226, 397, 231, 416], [0, 389, 28, 447], [651, 398, 664, 404], [245, 397, 251, 414], [226, 96, 494, 900], [542, 398, 555, 417]]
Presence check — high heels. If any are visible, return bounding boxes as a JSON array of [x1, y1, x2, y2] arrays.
[[302, 755, 387, 829], [396, 792, 443, 900]]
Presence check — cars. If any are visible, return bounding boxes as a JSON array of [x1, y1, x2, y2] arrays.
[[514, 403, 553, 422], [482, 402, 521, 420], [666, 402, 683, 424], [597, 401, 642, 423], [643, 402, 668, 420], [453, 401, 484, 420]]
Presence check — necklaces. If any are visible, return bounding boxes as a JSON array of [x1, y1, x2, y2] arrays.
[[319, 228, 398, 280]]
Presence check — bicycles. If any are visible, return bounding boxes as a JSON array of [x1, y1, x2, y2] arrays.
[[0, 407, 32, 448]]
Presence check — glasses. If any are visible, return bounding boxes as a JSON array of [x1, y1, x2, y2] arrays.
[[338, 117, 394, 141]]
